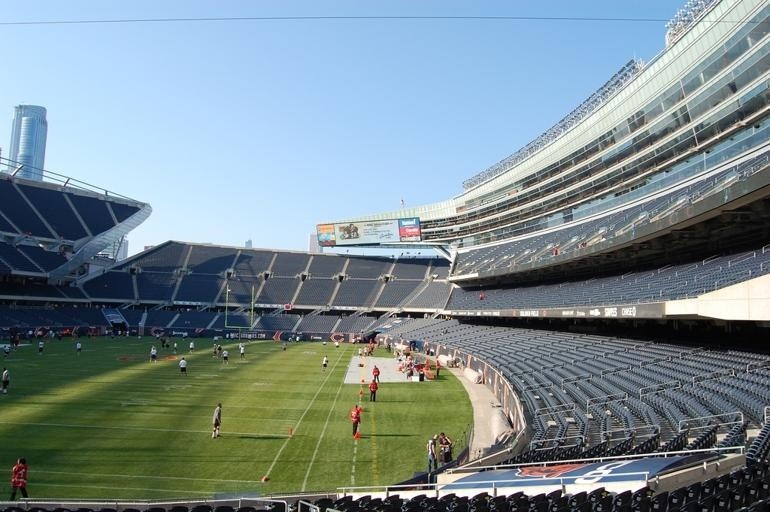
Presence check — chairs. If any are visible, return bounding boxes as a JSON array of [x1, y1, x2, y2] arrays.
[[384, 150, 770, 459], [0, 169, 452, 347], [0, 462, 770, 512]]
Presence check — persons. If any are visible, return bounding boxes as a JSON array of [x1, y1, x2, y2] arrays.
[[1, 327, 442, 403], [212, 403, 222, 439], [426, 434, 439, 472], [10, 456, 28, 501], [351, 406, 361, 437], [438, 432, 452, 462]]
